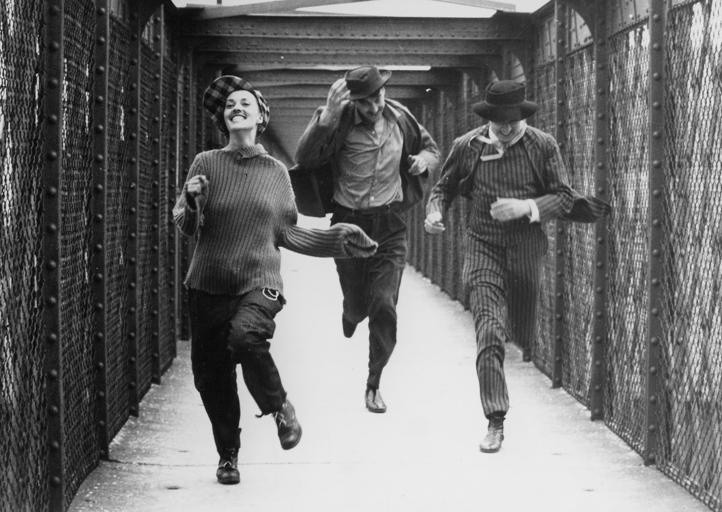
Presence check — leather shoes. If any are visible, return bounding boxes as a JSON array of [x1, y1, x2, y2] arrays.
[[363, 386, 386, 413], [214, 445, 244, 484], [274, 397, 303, 452], [478, 416, 506, 453], [341, 314, 357, 339]]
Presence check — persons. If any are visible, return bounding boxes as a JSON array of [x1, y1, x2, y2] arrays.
[[423, 78, 614, 454], [169, 74, 380, 485], [285, 65, 442, 413]]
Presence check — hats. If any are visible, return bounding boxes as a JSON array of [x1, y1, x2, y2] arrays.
[[468, 78, 541, 123], [200, 72, 272, 139], [339, 63, 393, 102]]
